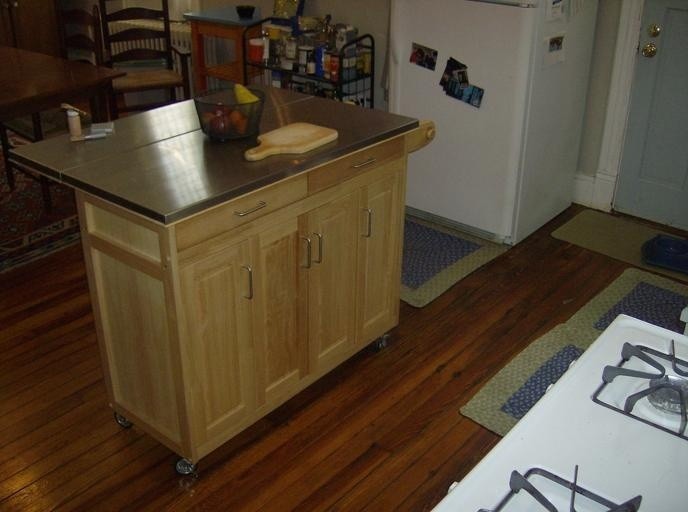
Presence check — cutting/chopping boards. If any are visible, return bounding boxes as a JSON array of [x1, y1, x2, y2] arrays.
[[243, 119, 340, 163]]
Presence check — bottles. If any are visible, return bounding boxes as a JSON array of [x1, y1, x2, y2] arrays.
[[249, 38, 265, 63], [66, 109, 82, 138], [306, 55, 318, 74], [263, 23, 292, 42], [284, 37, 298, 58]]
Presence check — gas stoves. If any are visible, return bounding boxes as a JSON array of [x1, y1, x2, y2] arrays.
[[433, 310, 687, 512]]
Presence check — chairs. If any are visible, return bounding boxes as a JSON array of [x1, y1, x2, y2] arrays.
[[99, 0, 191, 122]]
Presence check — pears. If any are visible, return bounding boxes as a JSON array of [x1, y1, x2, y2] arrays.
[[234, 84, 261, 116]]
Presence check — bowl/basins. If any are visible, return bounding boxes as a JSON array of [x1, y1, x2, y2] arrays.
[[235, 4, 256, 19]]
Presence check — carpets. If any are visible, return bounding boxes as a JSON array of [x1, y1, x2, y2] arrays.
[[551, 209, 688, 281], [400, 213, 512, 309], [0, 103, 82, 276], [460, 268, 688, 439]]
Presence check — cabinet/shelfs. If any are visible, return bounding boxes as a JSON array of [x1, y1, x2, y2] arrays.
[[256, 191, 355, 422], [354, 168, 406, 351], [174, 237, 259, 464], [242, 16, 375, 108]]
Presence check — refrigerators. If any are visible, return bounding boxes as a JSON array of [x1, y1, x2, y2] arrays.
[[384, 0, 600, 250]]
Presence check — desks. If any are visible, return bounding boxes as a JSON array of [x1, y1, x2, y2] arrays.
[[183, 7, 264, 96], [0, 46, 127, 210], [10, 80, 437, 479]]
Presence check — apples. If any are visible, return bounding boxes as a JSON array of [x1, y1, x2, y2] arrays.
[[200, 100, 247, 137]]
